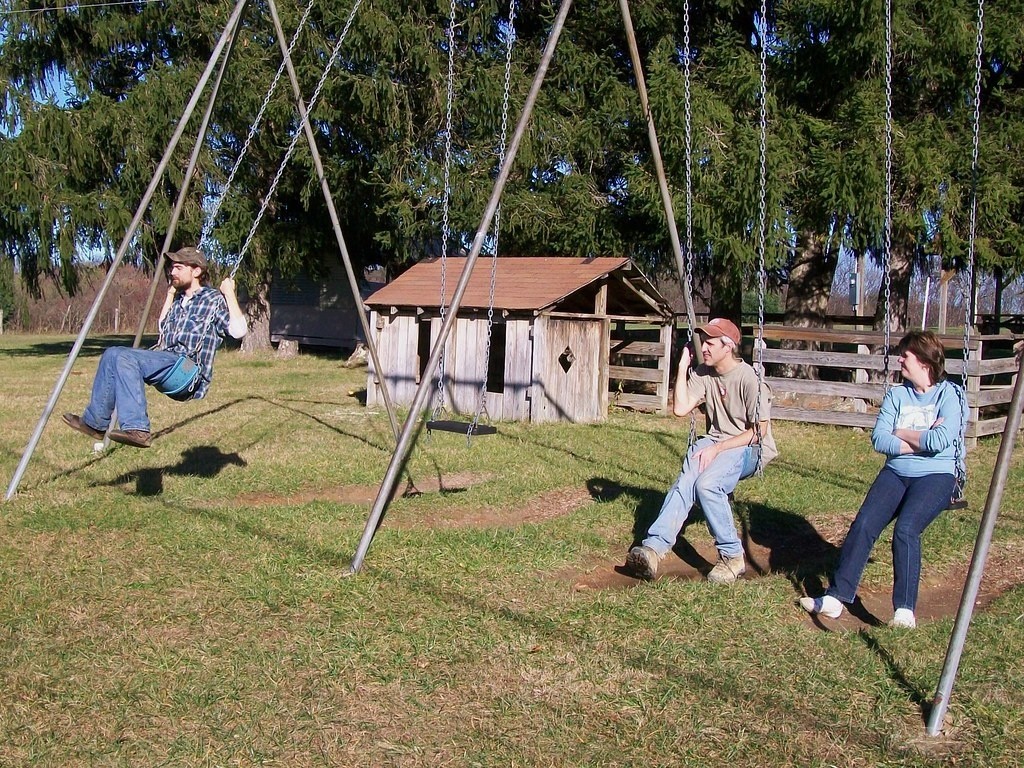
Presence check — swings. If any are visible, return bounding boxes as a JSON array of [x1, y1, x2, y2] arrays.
[[425, 0, 515, 436], [877, 0, 984, 511], [155, 0, 361, 395], [673, 0, 766, 481]]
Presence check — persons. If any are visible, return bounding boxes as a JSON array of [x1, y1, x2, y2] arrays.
[[800, 332, 970, 629], [62, 247, 247, 447], [627, 318, 779, 584]]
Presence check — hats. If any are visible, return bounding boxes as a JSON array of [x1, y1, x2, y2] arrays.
[[694, 318, 740, 344], [163, 247, 207, 269]]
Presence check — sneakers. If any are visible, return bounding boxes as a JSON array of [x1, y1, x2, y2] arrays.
[[707, 553, 745, 583], [626, 545, 661, 581]]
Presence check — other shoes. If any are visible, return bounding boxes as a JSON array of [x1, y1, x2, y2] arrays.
[[889, 615, 916, 629], [107, 429, 151, 448], [799, 596, 843, 618], [61, 413, 106, 440]]
